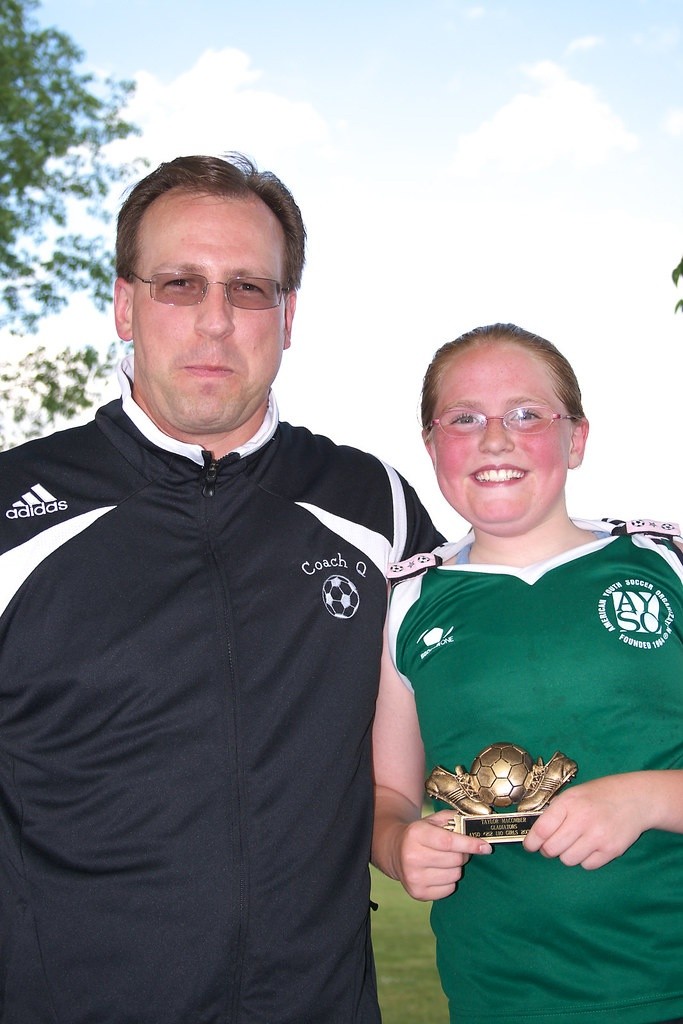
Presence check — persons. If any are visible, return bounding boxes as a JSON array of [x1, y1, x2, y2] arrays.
[[369, 322, 682, 1024], [0, 155, 449, 1024]]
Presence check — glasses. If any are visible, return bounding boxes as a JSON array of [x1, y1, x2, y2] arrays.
[[131, 271, 290, 310], [425, 406, 581, 439]]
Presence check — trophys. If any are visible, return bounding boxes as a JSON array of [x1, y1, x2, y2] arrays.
[[426, 741, 578, 849]]
